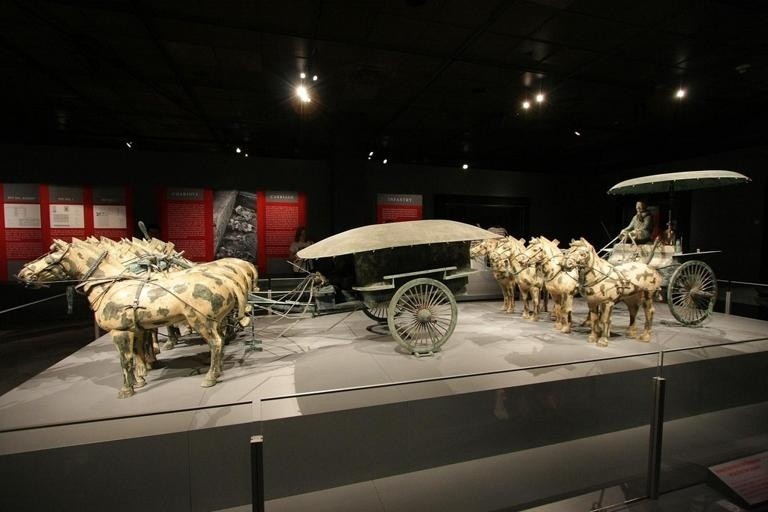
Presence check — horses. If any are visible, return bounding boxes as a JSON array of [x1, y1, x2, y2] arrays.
[[17, 236, 260, 399], [469, 234, 664, 347]]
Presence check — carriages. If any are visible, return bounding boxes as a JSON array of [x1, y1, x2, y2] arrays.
[[468, 169, 752, 345], [16, 220, 506, 398]]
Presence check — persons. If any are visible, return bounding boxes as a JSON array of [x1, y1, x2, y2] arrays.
[[619, 199, 655, 242], [288, 226, 319, 279]]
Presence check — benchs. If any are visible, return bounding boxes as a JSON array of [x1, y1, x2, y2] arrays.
[[608, 239, 682, 274]]
